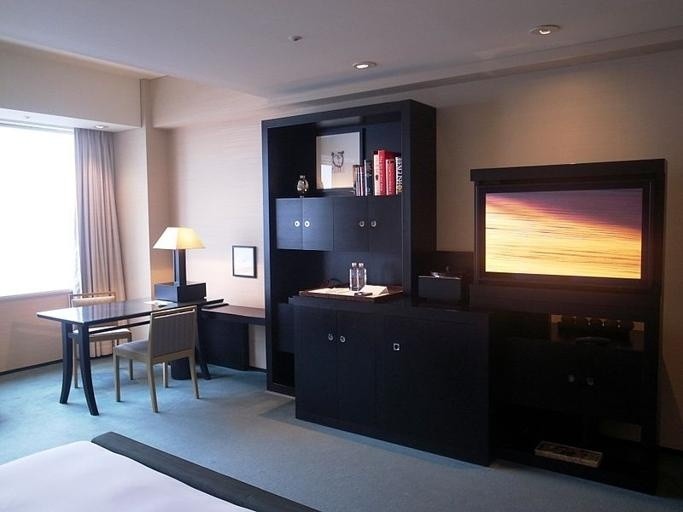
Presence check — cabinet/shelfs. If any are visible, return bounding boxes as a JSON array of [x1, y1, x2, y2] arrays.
[[376, 305, 488, 467], [261, 100, 436, 397], [295, 307, 375, 438]]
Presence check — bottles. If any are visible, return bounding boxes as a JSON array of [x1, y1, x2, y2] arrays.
[[356, 262, 367, 291], [349, 262, 358, 291]]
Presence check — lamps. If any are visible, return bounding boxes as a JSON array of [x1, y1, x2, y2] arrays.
[[152, 227, 207, 302]]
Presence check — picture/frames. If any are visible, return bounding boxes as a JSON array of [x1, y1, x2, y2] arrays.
[[232, 245, 256, 278]]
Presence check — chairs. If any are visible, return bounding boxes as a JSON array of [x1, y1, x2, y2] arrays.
[[69, 292, 134, 388], [113, 305, 200, 414]]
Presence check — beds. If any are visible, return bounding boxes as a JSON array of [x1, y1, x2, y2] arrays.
[[0, 432, 322, 512]]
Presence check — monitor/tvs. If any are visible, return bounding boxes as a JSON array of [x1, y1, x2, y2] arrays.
[[474, 179, 654, 291]]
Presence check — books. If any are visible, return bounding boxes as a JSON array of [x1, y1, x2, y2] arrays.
[[350, 147, 403, 198]]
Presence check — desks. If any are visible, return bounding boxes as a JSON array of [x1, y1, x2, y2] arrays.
[[200, 305, 265, 371], [37, 299, 211, 416]]
